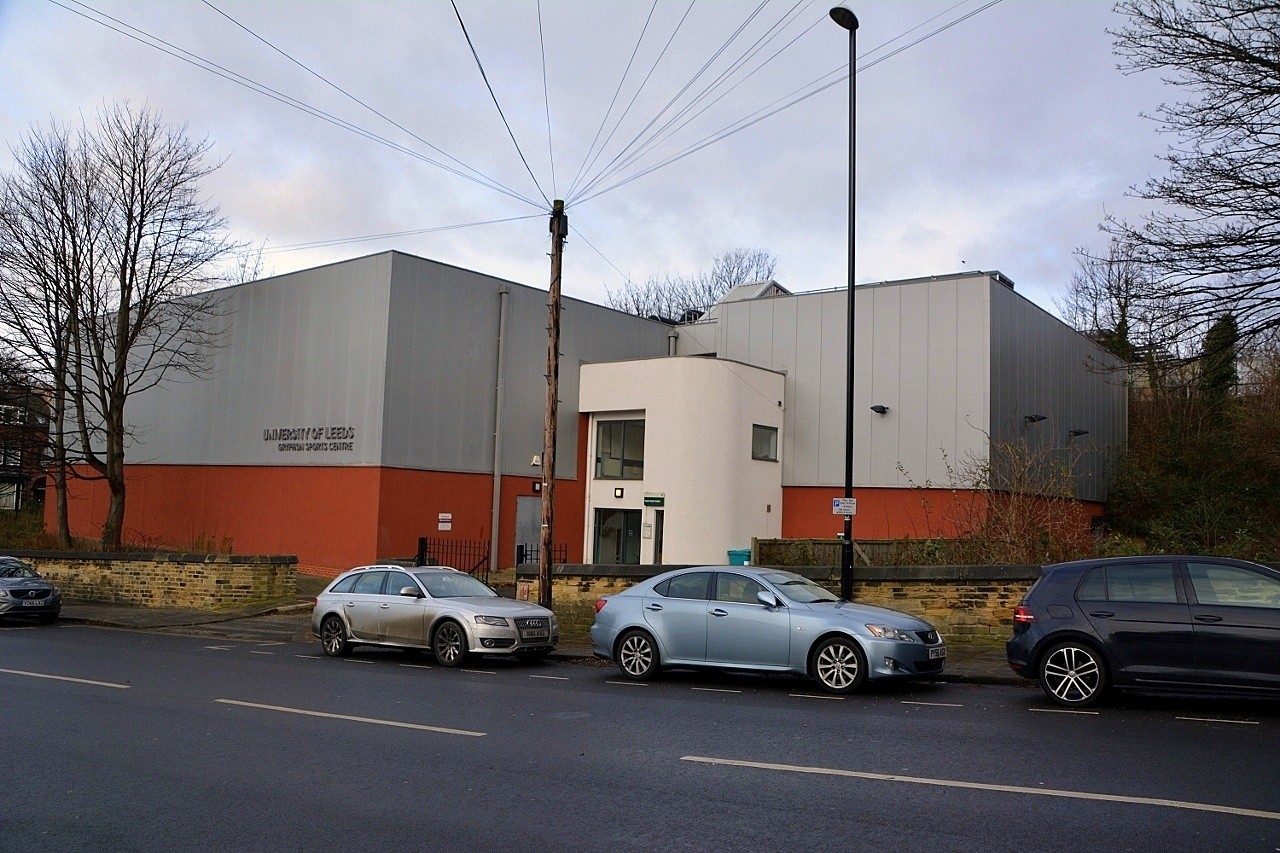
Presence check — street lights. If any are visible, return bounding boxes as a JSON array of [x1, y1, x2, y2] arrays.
[[829, 4, 859, 598]]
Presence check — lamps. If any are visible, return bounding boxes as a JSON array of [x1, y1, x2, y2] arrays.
[[1025, 414, 1047, 426], [870, 405, 889, 414], [1069, 429, 1089, 437]]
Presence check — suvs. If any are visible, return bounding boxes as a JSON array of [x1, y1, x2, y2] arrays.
[[312, 565, 559, 668], [1006, 555, 1280, 708]]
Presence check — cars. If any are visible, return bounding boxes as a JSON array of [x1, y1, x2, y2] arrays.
[[590, 565, 947, 696], [0, 555, 62, 627]]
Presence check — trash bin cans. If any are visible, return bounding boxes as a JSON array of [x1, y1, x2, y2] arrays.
[[727, 549, 751, 566]]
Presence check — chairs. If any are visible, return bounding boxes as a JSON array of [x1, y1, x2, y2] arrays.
[[744, 584, 761, 604], [362, 582, 377, 594]]
